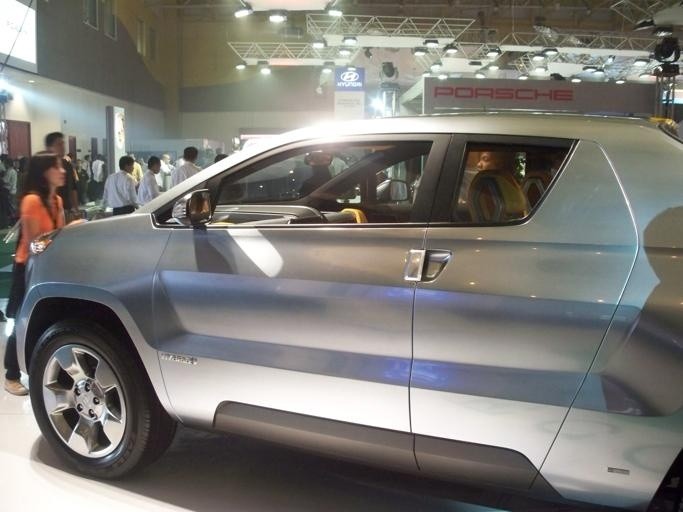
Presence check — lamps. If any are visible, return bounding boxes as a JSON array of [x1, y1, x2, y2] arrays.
[[232, 7, 681, 85]]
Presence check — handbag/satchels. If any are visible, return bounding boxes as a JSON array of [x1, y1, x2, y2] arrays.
[[79, 169, 89, 181]]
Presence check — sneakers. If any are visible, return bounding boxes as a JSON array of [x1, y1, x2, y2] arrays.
[[4, 379, 29, 396]]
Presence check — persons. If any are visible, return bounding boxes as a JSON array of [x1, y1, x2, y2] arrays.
[[0, 133, 229, 230], [470, 148, 527, 222], [3, 151, 89, 395]]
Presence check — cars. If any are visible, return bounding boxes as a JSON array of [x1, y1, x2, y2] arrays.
[[14, 110, 683, 511]]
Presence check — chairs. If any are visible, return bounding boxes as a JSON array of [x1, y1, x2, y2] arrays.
[[467, 169, 552, 225]]
